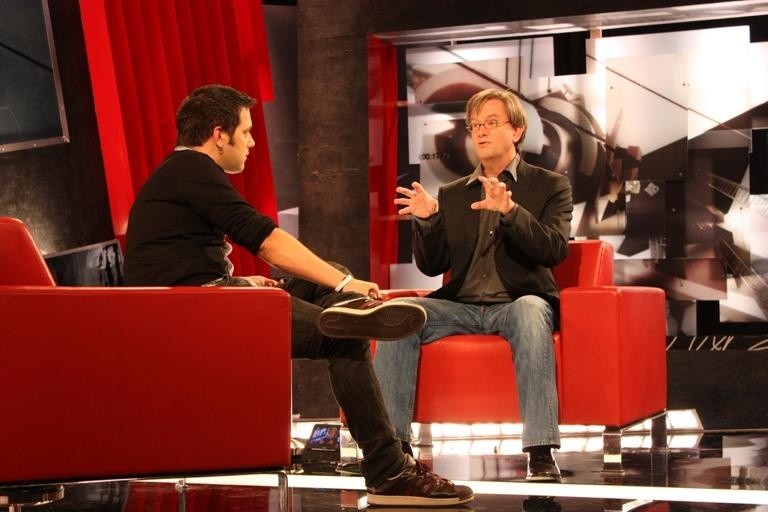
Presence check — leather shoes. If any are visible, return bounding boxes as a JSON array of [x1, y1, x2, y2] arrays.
[[525, 449, 563, 482], [336, 444, 414, 475]]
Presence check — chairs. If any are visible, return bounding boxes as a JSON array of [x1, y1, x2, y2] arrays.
[[1, 212, 292, 488], [339, 240, 669, 469]]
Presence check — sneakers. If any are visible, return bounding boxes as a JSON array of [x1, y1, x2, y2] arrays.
[[317, 297, 427, 341], [366, 452, 474, 506]]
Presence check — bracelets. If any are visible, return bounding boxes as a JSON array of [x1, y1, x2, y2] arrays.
[[333, 273, 353, 293]]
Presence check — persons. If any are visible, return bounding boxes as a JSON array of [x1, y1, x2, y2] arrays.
[[120, 82, 477, 509], [332, 85, 576, 484], [318, 427, 339, 448]]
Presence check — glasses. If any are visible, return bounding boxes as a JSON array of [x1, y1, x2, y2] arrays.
[[466, 119, 511, 132]]
